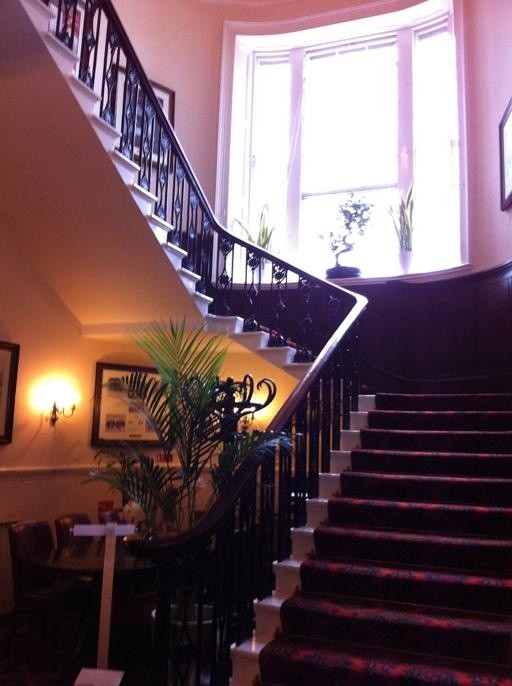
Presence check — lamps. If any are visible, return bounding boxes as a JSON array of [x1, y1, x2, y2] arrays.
[[28, 374, 81, 429]]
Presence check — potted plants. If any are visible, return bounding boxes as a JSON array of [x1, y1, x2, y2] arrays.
[[233, 203, 275, 282], [107, 310, 241, 663], [326, 198, 371, 278], [393, 183, 417, 270]]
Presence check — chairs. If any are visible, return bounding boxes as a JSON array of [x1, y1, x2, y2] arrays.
[[7, 501, 231, 677]]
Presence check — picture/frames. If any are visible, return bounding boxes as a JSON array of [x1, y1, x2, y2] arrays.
[[500, 96, 512, 211], [49, 1, 90, 81], [0, 339, 21, 446], [108, 61, 178, 174], [91, 362, 179, 449]]
